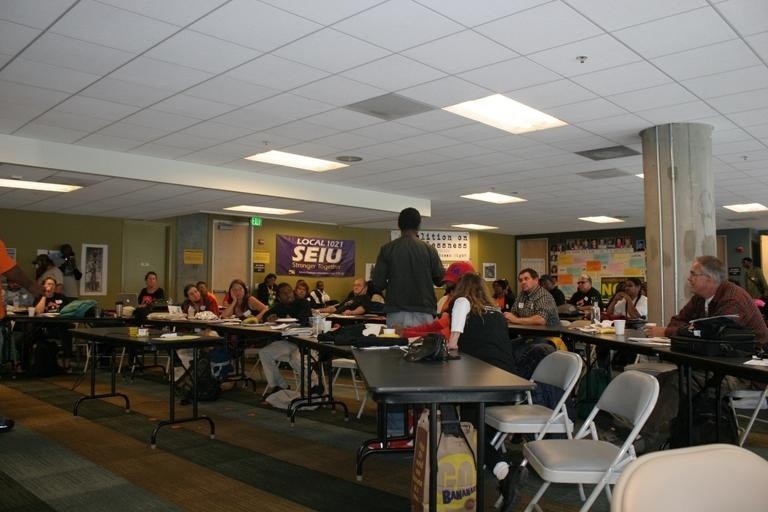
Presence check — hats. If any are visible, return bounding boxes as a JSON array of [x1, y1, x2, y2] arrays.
[[32, 254, 49, 264], [441, 259, 478, 285]]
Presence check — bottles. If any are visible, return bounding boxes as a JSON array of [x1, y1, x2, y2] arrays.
[[114, 300, 125, 318], [590, 300, 601, 326], [188, 305, 195, 320]]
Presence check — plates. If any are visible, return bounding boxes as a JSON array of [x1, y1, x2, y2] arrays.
[[274, 317, 299, 324], [14, 310, 28, 315]]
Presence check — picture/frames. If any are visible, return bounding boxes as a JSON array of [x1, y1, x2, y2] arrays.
[[79, 242, 109, 297]]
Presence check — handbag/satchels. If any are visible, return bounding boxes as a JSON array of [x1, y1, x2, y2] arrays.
[[669, 318, 761, 357], [403, 334, 452, 365]]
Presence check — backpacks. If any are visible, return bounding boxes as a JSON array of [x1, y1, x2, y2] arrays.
[[60, 298, 98, 318], [671, 397, 741, 449]]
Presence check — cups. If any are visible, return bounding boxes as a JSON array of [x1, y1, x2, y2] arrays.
[[383, 327, 395, 336], [312, 317, 332, 337], [128, 326, 149, 337], [645, 322, 657, 338], [613, 319, 626, 335], [28, 307, 35, 317]]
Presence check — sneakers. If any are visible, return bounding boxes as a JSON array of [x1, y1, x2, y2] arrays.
[[0, 415, 16, 434], [497, 466, 529, 511]]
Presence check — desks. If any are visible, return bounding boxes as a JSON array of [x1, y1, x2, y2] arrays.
[[352, 344, 537, 511], [510, 323, 768, 445], [1, 316, 387, 450]]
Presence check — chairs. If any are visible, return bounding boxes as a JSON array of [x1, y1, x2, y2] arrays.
[[484, 350, 661, 511], [612, 443, 768, 512]]
